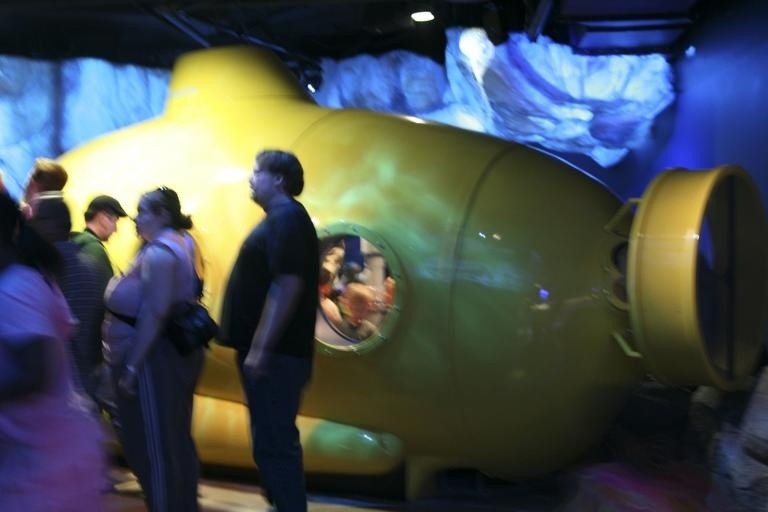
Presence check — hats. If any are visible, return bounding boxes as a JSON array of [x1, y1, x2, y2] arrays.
[[89, 195, 128, 218]]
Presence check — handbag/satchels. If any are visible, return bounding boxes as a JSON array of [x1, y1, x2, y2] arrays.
[[163, 300, 219, 359]]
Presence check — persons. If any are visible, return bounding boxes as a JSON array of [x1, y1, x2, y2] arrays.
[[216, 146, 321, 511], [69, 194, 128, 282], [97, 184, 217, 511], [21, 190, 106, 395], [0, 189, 109, 511], [0, 155, 69, 209]]
